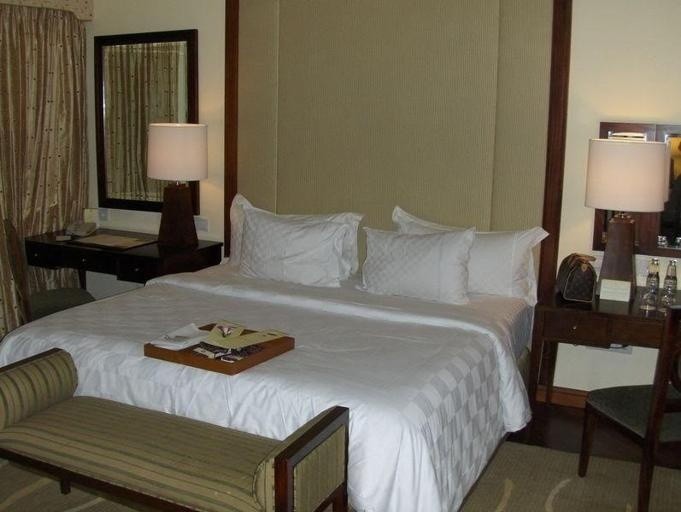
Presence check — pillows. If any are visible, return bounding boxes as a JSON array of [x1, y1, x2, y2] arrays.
[[355, 225, 477, 306], [237, 205, 351, 290], [391, 205, 552, 306], [229, 193, 366, 278]]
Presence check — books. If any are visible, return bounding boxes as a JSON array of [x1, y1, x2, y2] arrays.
[[144, 318, 295, 377]]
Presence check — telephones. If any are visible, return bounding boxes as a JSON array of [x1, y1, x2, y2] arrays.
[[65, 220, 97, 237]]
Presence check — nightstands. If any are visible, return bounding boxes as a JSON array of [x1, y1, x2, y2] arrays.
[[115, 235, 224, 291], [527, 287, 681, 445]]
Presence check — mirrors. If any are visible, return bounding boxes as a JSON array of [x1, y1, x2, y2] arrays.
[[591, 121, 681, 258], [94, 29, 201, 214]]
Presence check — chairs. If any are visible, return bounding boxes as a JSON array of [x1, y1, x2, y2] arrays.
[[4, 216, 96, 326], [579, 308, 681, 511]]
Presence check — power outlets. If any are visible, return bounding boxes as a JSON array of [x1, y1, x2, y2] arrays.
[[609, 342, 633, 354]]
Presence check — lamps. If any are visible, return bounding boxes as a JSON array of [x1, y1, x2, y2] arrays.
[[147, 123, 209, 249], [585, 139, 668, 301]]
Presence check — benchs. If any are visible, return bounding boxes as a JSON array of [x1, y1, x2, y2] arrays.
[[0, 347, 351, 512]]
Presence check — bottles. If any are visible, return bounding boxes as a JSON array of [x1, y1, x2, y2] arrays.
[[660, 286, 676, 312], [646, 257, 662, 288], [663, 259, 678, 287], [638, 284, 657, 311]]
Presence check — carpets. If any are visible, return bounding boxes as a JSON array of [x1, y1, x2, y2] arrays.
[[459, 441, 681, 512]]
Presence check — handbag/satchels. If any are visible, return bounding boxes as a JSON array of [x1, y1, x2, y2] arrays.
[[557, 254, 597, 302]]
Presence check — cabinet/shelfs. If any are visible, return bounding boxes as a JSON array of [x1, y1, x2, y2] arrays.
[[22, 220, 158, 298]]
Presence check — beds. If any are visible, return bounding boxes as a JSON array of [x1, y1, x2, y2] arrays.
[[0, 253, 549, 510]]
